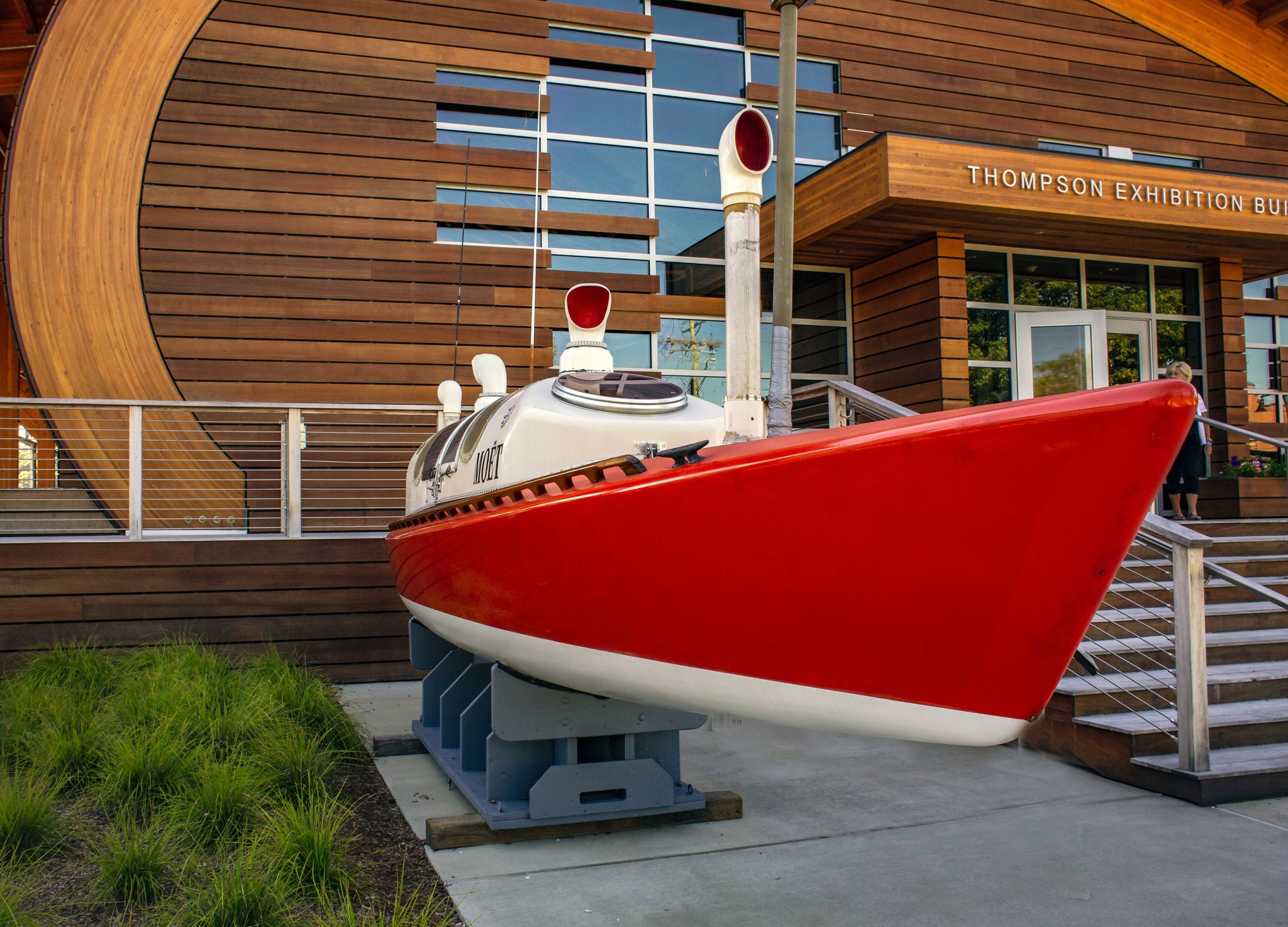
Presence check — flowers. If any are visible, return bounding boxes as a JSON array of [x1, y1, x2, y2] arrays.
[[1205, 455, 1265, 477]]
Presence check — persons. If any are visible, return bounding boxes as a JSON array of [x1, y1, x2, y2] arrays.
[[1164, 362, 1212, 521]]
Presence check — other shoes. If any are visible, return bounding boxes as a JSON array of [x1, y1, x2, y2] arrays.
[[1187, 513, 1201, 520], [1173, 514, 1185, 520]]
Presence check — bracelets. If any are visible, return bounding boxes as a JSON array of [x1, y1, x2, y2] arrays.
[[1206, 438, 1212, 442], [1206, 442, 1212, 445]]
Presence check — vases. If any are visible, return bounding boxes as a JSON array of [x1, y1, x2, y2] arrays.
[[1197, 477, 1288, 517]]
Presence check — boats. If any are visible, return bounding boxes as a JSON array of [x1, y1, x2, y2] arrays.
[[385, 282, 1197, 746]]
